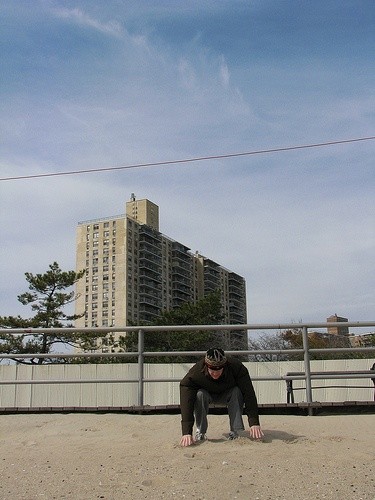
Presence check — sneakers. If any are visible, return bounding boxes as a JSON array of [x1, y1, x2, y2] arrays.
[[228, 431, 237, 440], [195, 428, 206, 441]]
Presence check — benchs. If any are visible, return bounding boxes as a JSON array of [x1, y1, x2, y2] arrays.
[[286, 370, 375, 403]]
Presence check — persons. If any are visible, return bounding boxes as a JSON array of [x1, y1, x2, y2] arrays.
[[178, 345, 266, 447]]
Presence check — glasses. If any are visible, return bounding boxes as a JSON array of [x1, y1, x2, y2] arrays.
[[208, 366, 224, 370]]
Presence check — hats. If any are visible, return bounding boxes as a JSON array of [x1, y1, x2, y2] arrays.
[[205, 348, 227, 366]]
